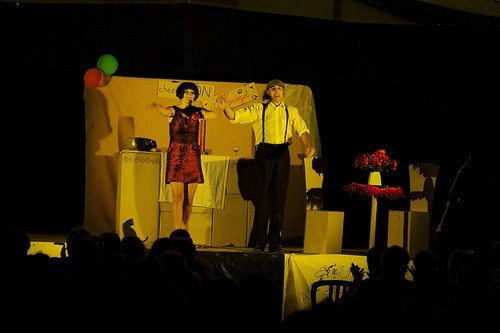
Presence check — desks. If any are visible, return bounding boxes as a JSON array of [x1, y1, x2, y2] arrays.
[[343, 182, 405, 250]]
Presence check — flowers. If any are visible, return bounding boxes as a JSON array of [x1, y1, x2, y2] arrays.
[[355, 149, 398, 172]]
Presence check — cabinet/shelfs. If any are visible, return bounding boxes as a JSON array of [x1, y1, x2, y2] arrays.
[[115, 149, 281, 251]]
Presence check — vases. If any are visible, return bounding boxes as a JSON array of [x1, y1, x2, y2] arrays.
[[367, 171, 383, 187]]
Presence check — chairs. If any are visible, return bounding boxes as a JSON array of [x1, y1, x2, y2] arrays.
[[303, 154, 345, 254], [311, 280, 353, 308], [387, 163, 439, 259]]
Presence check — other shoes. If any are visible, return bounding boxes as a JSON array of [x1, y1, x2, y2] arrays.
[[255, 245, 263, 252], [269, 245, 281, 252]]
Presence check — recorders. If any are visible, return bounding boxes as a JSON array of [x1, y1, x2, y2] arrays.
[[128, 136, 158, 151]]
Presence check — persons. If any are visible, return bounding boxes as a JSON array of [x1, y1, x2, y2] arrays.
[[149, 82, 224, 229], [216, 79, 315, 250], [0, 227, 500, 333]]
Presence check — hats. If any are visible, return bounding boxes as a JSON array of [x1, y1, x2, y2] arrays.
[[267, 80, 285, 89]]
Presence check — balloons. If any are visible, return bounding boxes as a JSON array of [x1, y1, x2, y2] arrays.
[[84, 68, 103, 88], [97, 54, 119, 76]]
